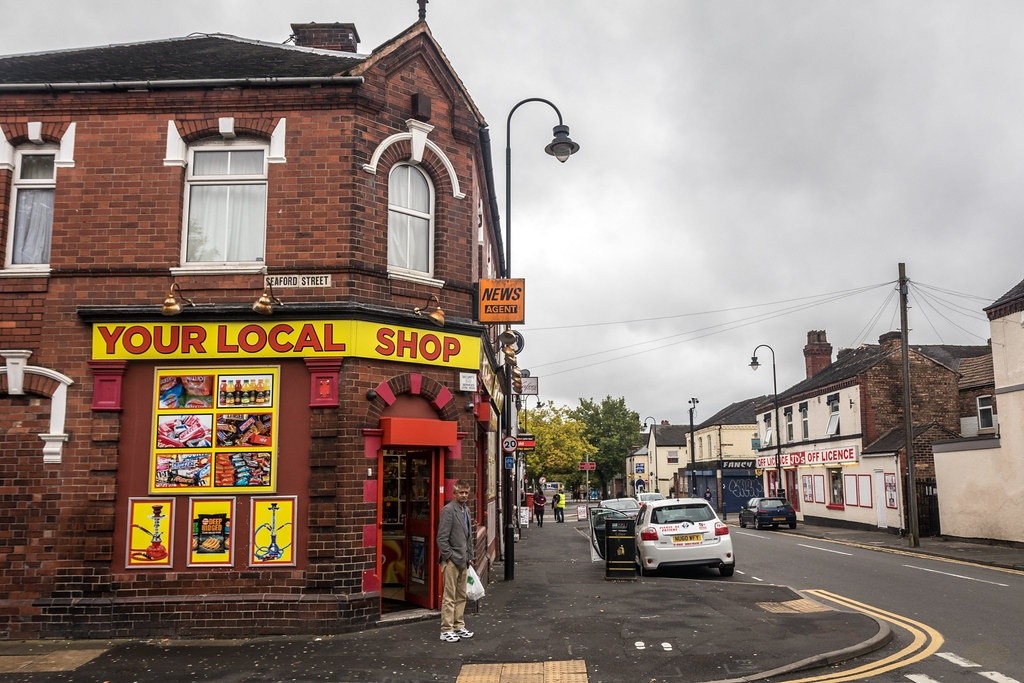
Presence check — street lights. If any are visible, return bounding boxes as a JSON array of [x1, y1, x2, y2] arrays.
[[524, 394, 542, 492], [748, 344, 782, 497], [506, 99, 580, 580], [643, 417, 658, 493]]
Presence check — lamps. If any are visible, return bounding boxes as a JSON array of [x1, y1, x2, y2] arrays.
[[485, 321, 522, 393], [414, 294, 445, 327], [251, 282, 284, 314], [162, 282, 195, 315]]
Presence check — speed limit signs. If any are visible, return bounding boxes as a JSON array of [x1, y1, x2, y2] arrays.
[[502, 436, 518, 453]]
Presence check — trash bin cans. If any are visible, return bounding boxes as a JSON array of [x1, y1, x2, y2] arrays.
[[604, 518, 637, 581]]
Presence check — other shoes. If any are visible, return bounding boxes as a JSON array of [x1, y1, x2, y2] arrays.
[[540, 523, 543, 527], [538, 521, 540, 526], [556, 521, 560, 523], [560, 520, 564, 523]]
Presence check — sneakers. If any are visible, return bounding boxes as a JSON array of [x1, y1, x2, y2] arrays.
[[455, 627, 474, 638], [440, 631, 460, 643]]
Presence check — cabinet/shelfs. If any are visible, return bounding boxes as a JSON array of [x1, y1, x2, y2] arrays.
[[383, 453, 429, 526]]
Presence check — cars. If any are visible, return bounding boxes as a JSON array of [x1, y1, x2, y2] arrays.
[[591, 498, 641, 530], [633, 493, 668, 510], [738, 497, 797, 530], [588, 498, 735, 577]]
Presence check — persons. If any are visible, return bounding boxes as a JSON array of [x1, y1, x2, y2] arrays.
[[436, 480, 474, 642], [521, 487, 566, 527], [704, 487, 713, 504]]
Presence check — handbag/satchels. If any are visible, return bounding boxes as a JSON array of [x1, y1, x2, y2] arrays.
[[465, 564, 485, 601]]
